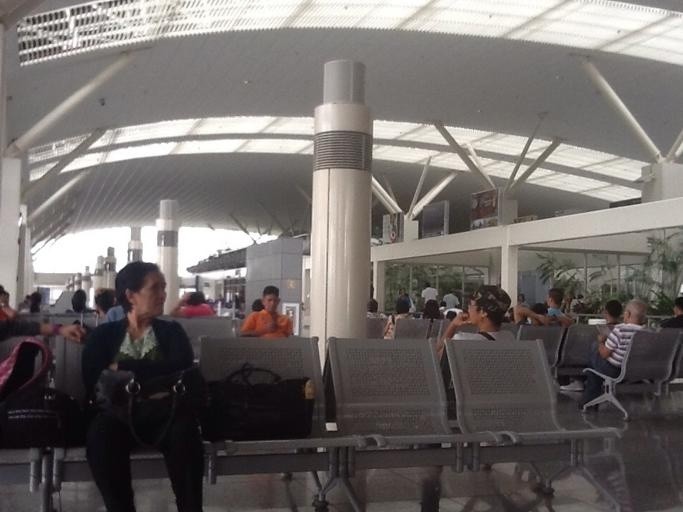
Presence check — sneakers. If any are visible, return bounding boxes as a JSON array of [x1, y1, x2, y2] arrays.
[[559, 381, 584, 391]]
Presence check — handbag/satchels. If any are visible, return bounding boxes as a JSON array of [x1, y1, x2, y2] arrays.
[[0, 388, 80, 449], [94, 365, 197, 449], [199, 363, 314, 441]]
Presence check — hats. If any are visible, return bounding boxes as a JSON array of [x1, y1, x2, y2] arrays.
[[471, 285, 510, 312]]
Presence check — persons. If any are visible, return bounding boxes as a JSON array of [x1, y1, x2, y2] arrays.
[[659, 296, 683, 330], [171, 292, 214, 319], [1, 290, 119, 349], [240, 286, 291, 340], [252, 300, 264, 311], [587, 455, 629, 512], [80, 261, 204, 511], [370, 283, 463, 340], [509, 288, 590, 327], [578, 300, 647, 411], [604, 300, 622, 325], [435, 284, 515, 385]]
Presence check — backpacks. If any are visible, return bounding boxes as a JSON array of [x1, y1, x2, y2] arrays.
[[0, 339, 51, 394]]
